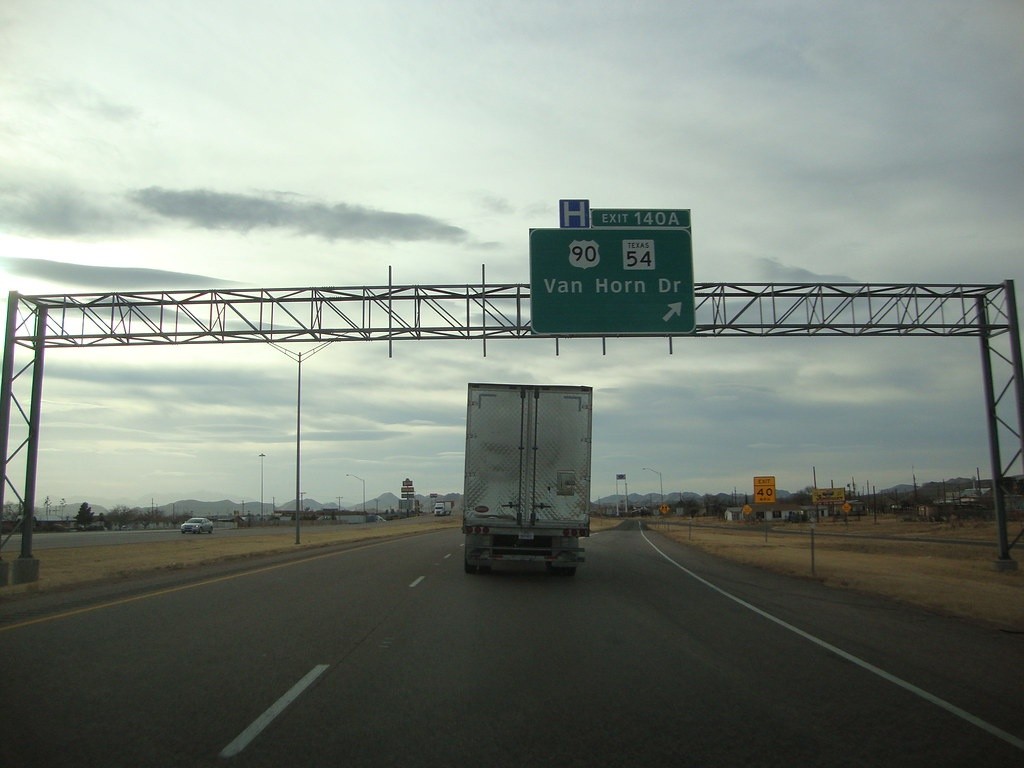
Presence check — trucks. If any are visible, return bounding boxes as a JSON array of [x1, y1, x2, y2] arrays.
[[434, 501, 452, 516], [462, 382, 593, 579]]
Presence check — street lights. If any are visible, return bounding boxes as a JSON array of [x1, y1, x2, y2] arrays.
[[346, 474, 365, 513], [642, 468, 663, 515], [259, 453, 266, 527]]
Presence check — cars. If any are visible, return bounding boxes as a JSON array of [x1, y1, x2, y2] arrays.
[[46, 524, 71, 532], [181, 518, 213, 534], [86, 523, 105, 531]]
